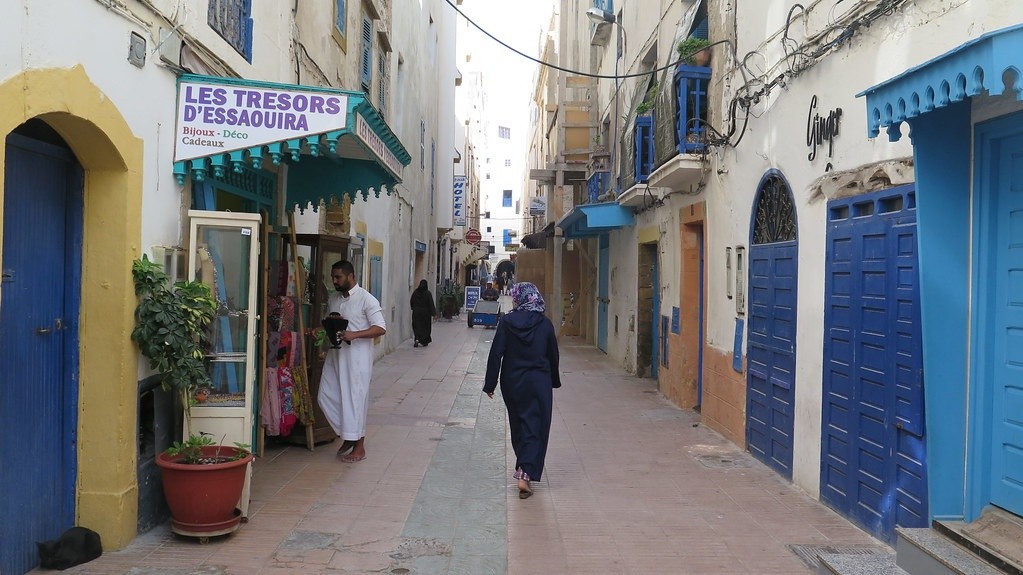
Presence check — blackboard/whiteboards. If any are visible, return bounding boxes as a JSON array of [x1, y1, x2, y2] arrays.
[[465, 286, 480, 311]]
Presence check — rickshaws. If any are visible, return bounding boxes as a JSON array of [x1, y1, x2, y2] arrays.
[[467, 298, 505, 327]]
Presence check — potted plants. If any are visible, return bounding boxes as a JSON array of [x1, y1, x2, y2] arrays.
[[677, 36, 711, 66], [154, 432, 253, 532], [592, 135, 606, 152], [635, 84, 658, 117], [439, 280, 465, 319]]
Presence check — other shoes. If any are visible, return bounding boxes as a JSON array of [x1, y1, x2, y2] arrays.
[[414, 340, 418, 347], [422, 343, 428, 347]]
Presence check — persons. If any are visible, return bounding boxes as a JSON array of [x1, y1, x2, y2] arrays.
[[482, 282, 561, 500], [497, 271, 513, 295], [315, 259, 386, 463], [409, 279, 437, 348], [483, 283, 499, 329]]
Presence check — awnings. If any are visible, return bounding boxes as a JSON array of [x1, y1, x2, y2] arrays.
[[173, 72, 412, 215]]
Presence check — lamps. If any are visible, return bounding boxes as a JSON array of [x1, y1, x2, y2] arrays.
[[585, 7, 627, 56]]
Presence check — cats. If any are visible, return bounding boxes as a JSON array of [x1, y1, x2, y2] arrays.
[[36, 527, 105, 568]]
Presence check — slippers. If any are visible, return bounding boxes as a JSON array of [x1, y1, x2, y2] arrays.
[[343, 453, 368, 463], [337, 441, 353, 455], [519, 487, 532, 498]]
